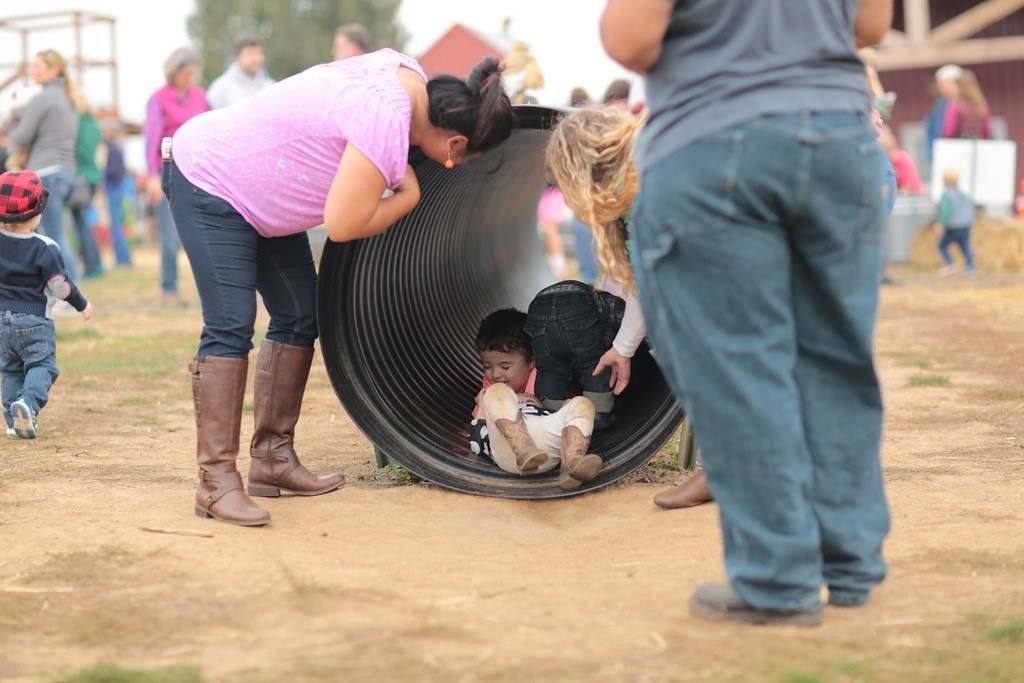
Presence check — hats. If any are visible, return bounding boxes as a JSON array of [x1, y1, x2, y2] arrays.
[[0, 171, 49, 222], [937, 66, 963, 82]]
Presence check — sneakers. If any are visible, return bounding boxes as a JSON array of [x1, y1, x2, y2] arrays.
[[652, 460, 712, 510], [5, 427, 18, 436], [827, 584, 872, 606], [10, 396, 38, 439], [692, 580, 825, 626]]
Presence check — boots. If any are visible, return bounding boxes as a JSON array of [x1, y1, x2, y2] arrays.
[[189, 354, 272, 525], [494, 408, 549, 477], [557, 425, 603, 491], [247, 338, 347, 497]]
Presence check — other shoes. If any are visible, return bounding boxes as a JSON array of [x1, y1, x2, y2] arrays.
[[52, 296, 89, 316], [593, 406, 619, 428]]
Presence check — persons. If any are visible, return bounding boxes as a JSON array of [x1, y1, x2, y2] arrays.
[[0, 170, 92, 439], [933, 169, 977, 277], [66, 89, 105, 280], [926, 64, 998, 164], [161, 45, 518, 528], [860, 47, 927, 230], [469, 307, 603, 489], [206, 34, 277, 111], [521, 79, 714, 510], [600, 1, 894, 620], [7, 47, 80, 317], [333, 23, 373, 60], [98, 118, 134, 265], [143, 47, 215, 311]]
